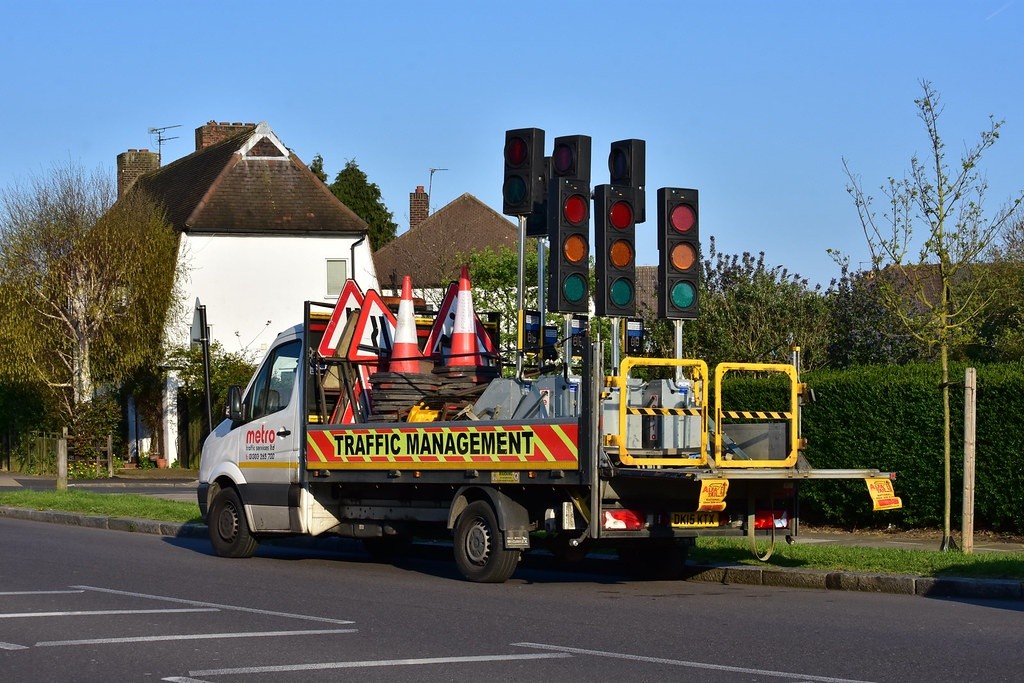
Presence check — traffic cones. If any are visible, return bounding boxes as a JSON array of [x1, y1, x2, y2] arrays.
[[371, 274, 432, 378], [433, 262, 497, 374]]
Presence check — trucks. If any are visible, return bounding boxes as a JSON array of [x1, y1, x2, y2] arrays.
[[198, 299, 896, 583]]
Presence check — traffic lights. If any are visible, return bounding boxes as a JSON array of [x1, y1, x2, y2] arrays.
[[552, 134, 592, 183], [610, 140, 646, 224], [658, 188, 700, 321], [503, 128, 546, 216], [594, 183, 638, 317], [548, 178, 591, 313]]
